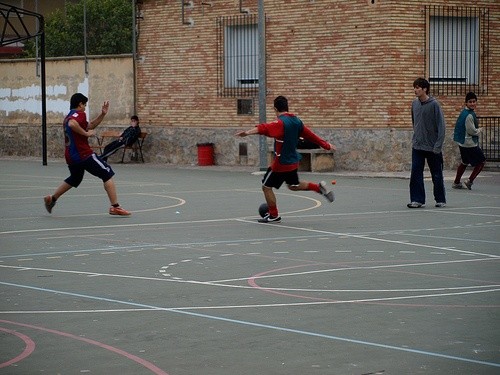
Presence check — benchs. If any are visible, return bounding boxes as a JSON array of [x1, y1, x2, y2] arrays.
[[268, 147, 336, 172], [89, 128, 147, 163]]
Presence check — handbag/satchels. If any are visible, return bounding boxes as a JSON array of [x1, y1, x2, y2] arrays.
[[297, 139, 320, 149]]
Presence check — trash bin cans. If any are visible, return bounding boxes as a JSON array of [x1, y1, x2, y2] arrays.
[[196, 142, 215, 166]]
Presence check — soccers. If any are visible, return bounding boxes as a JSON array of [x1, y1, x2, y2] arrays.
[[258, 203, 269, 218]]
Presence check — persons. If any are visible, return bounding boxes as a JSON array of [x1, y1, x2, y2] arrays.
[[98, 115, 142, 163], [407, 77, 447, 209], [232, 96, 336, 224], [43, 92, 132, 216], [451, 92, 486, 190]]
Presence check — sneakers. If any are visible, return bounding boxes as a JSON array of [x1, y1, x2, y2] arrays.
[[258, 214, 281, 223], [109, 206, 131, 215], [44, 195, 56, 213], [319, 181, 335, 203]]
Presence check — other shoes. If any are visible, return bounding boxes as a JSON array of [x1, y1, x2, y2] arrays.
[[464, 179, 474, 190], [407, 202, 425, 208], [452, 182, 468, 189], [435, 203, 446, 207]]
